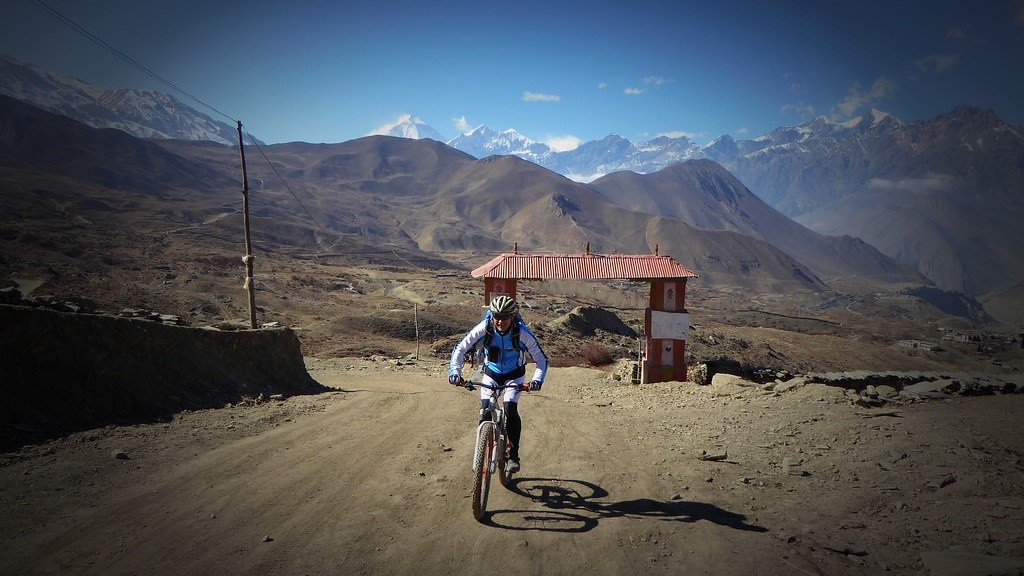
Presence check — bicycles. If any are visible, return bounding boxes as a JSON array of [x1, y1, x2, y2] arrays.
[[458, 377, 531, 521]]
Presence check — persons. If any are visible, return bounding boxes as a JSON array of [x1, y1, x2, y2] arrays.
[[449, 295, 548, 473]]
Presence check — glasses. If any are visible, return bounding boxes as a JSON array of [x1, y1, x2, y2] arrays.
[[492, 315, 512, 320]]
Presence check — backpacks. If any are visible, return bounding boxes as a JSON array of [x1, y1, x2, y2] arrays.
[[482, 310, 526, 353]]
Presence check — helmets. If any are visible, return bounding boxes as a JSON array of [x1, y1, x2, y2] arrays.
[[489, 296, 518, 315]]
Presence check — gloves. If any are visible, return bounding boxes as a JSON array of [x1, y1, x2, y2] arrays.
[[449, 374, 464, 384], [527, 379, 542, 392]]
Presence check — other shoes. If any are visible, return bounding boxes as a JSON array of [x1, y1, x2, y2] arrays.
[[507, 457, 520, 473]]
